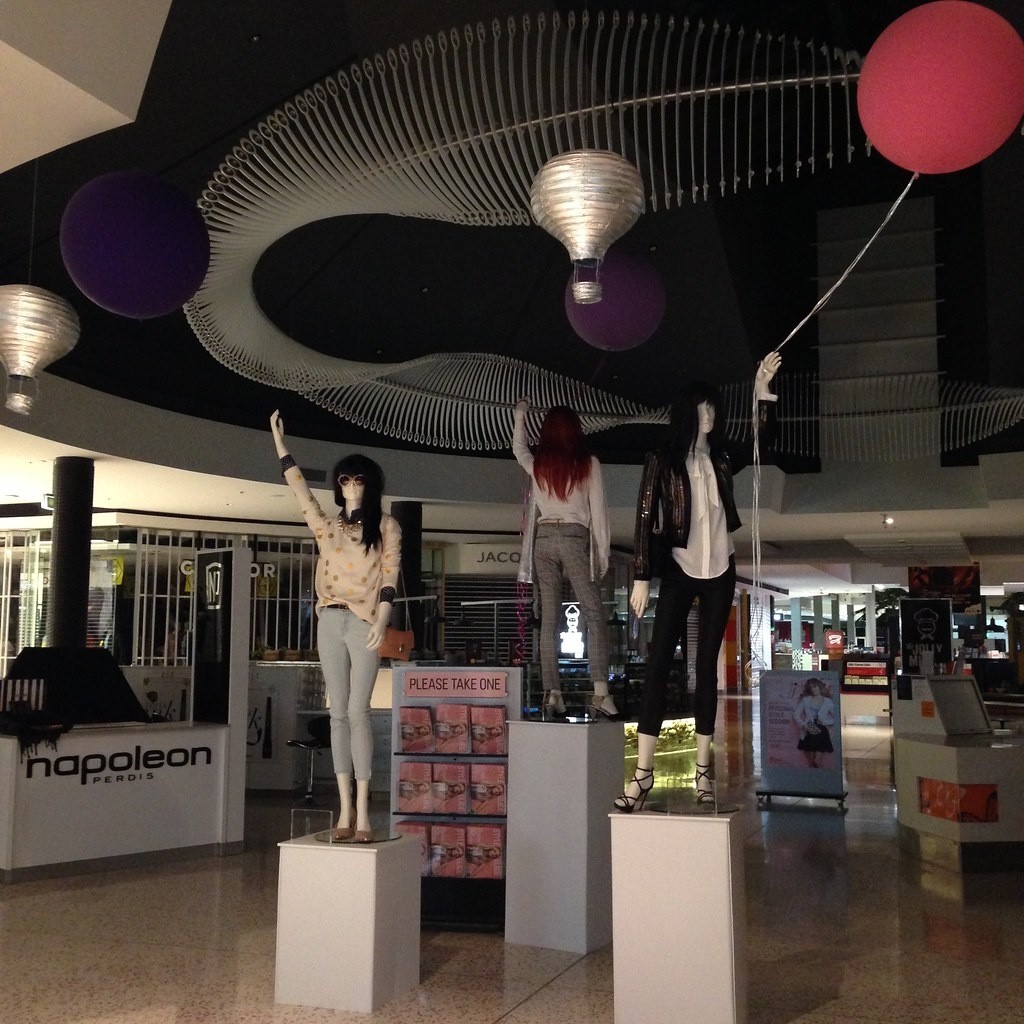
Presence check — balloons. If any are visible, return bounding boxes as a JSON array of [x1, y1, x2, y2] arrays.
[[857, 0, 1024, 174]]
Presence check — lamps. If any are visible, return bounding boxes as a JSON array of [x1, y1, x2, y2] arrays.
[[0, 156, 81, 416], [529, 0, 644, 305]]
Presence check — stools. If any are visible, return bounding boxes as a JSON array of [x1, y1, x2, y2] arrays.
[[287, 716, 330, 806]]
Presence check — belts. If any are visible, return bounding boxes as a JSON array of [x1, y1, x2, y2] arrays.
[[327, 604, 349, 609], [540, 519, 563, 524]]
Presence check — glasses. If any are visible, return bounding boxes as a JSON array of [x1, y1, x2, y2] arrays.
[[338, 474, 363, 486]]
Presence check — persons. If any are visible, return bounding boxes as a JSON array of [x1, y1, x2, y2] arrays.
[[613, 351, 782, 814], [271, 410, 403, 841], [513, 397, 628, 721], [794, 678, 834, 767]]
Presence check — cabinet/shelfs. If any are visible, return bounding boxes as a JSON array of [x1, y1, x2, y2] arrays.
[[541, 660, 690, 722]]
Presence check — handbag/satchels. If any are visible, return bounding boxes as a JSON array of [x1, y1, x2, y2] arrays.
[[648, 531, 673, 579], [377, 627, 414, 661]]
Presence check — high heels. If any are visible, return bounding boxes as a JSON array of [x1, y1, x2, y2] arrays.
[[335, 809, 356, 839], [588, 694, 632, 722], [546, 693, 570, 722], [614, 767, 655, 813], [355, 823, 372, 842], [695, 764, 715, 807]]
